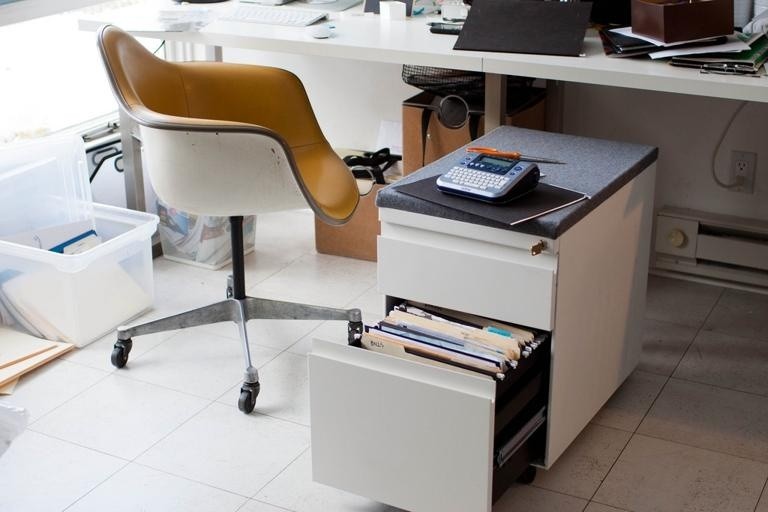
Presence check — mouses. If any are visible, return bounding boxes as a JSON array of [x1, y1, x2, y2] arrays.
[[312, 25, 334, 38]]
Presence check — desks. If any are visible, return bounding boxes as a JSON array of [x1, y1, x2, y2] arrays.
[[76, 5, 735, 262]]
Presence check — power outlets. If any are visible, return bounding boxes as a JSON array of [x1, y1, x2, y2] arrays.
[[727, 150, 757, 195]]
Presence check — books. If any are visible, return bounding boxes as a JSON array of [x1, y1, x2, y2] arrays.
[[0, 229, 152, 391], [357, 305, 535, 371], [393, 171, 588, 227], [598, 25, 766, 73]]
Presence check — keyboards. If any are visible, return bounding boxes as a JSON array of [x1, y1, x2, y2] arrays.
[[219, 3, 329, 27]]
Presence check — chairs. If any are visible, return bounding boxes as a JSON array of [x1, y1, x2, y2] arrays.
[[93, 23, 364, 417]]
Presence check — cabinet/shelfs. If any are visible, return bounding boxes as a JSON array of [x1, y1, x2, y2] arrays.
[[306, 125, 662, 511]]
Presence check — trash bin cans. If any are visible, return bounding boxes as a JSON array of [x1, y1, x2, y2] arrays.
[[155, 200, 256, 271]]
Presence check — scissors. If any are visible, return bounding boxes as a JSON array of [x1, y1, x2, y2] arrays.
[[466, 145, 565, 165]]
